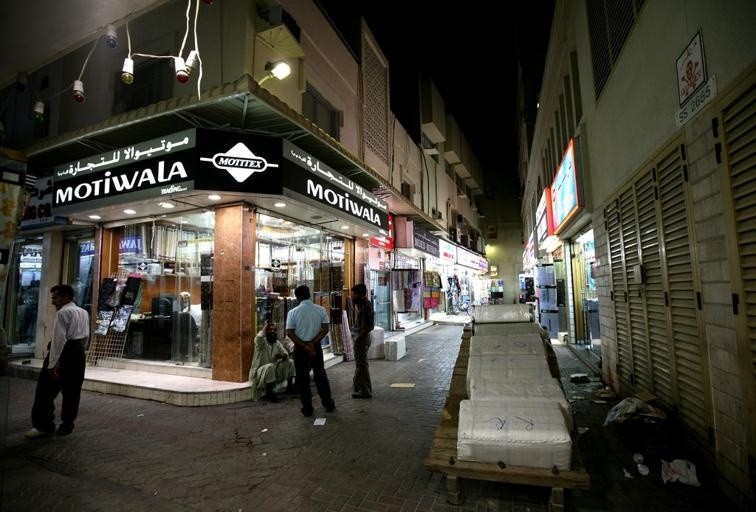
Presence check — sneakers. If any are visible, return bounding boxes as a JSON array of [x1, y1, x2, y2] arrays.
[[266, 392, 280, 403], [352, 391, 362, 398], [60, 422, 75, 439], [24, 427, 55, 437]]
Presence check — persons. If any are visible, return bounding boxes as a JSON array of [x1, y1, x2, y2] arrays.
[[286, 285, 335, 416], [407, 282, 421, 316], [249, 320, 297, 404], [24, 284, 90, 437], [349, 282, 376, 399]]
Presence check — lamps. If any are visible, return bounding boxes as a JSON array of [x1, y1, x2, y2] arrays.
[[105, 25, 120, 50], [31, 101, 43, 123], [257, 61, 293, 86], [120, 57, 135, 85], [173, 55, 190, 84], [74, 79, 85, 103], [184, 50, 198, 72]]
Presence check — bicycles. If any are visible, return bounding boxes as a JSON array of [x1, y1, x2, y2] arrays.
[[449, 300, 474, 317]]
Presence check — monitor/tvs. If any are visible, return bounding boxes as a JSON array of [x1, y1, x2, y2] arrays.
[[21, 272, 42, 287]]
